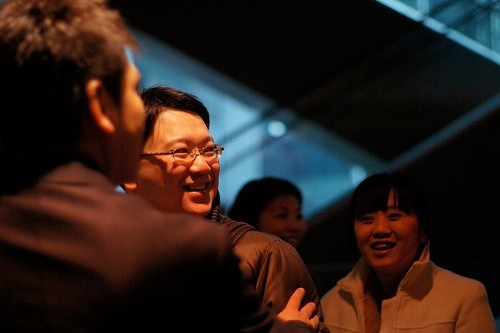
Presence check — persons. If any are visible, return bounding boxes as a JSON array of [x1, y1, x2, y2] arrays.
[[320, 172, 498, 333], [226, 176, 338, 301], [122, 86, 329, 333], [0, 0, 320, 333]]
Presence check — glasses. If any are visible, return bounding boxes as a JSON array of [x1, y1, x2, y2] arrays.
[[141, 144, 222, 161]]
